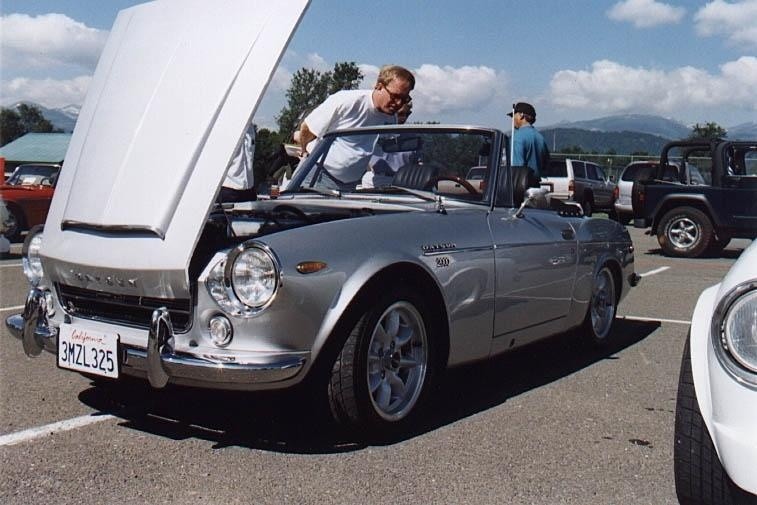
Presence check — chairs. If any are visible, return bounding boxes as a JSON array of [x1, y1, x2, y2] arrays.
[[484, 166, 535, 205], [726, 147, 735, 174], [391, 164, 439, 191]]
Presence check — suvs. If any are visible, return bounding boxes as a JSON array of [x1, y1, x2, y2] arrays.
[[630, 136, 756, 257], [539, 156, 615, 218]]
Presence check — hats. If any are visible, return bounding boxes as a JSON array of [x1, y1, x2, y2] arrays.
[[507, 103, 536, 117]]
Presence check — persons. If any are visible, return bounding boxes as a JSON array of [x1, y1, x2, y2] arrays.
[[212, 120, 258, 205], [362, 92, 413, 189], [285, 63, 416, 196], [504, 101, 551, 190]]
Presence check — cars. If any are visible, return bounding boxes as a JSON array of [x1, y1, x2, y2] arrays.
[[672, 238, 756, 505], [0, 162, 62, 241], [4, 0, 641, 439], [612, 160, 680, 224], [464, 165, 487, 194]]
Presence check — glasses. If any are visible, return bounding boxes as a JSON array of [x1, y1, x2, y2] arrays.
[[383, 85, 412, 104]]
